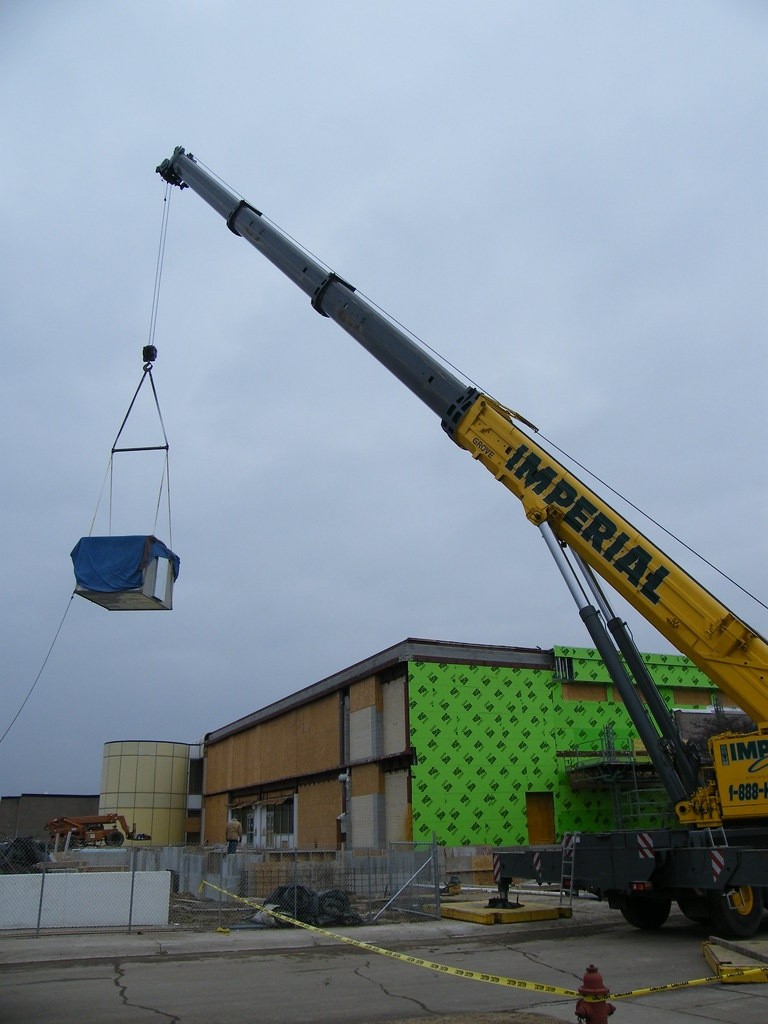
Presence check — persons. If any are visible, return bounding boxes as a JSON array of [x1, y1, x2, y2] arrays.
[[226, 815, 242, 854]]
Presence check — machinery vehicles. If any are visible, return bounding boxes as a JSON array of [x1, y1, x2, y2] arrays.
[[155, 144, 767, 940], [43, 813, 151, 846]]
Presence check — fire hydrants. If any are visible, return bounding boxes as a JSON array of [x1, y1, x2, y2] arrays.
[[575, 963, 618, 1024]]
